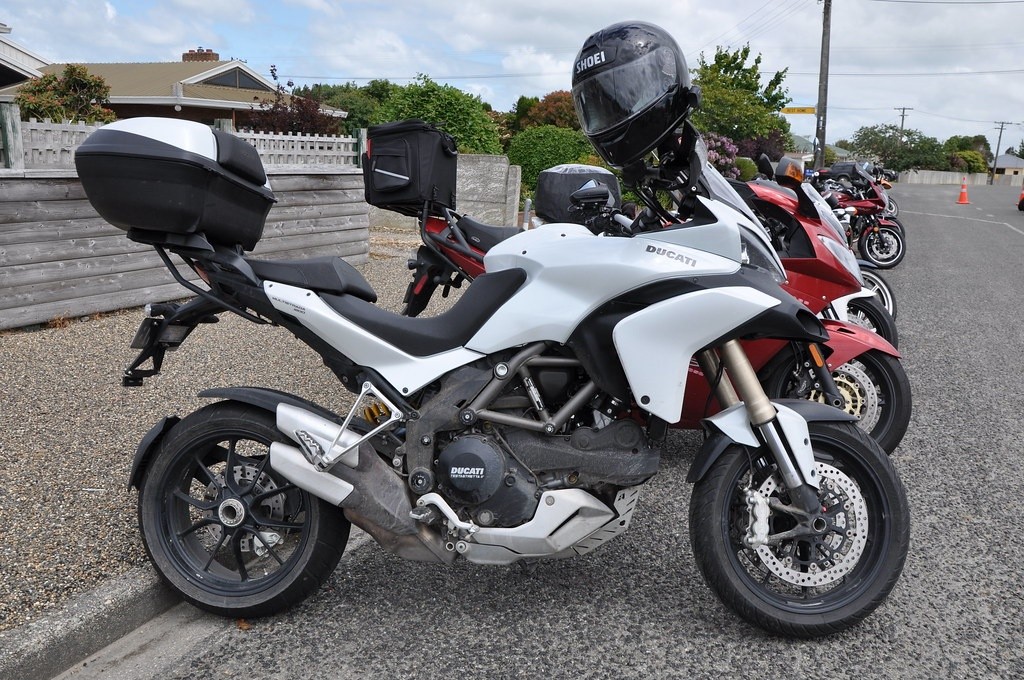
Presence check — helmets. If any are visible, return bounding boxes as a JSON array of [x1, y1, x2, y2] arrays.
[[570, 20, 699, 171]]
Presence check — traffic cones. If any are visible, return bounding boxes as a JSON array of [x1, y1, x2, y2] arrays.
[[1015, 178, 1024, 205], [955, 176, 970, 204]]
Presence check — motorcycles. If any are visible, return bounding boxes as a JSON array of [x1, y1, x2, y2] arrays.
[[359, 117, 912, 455], [73, 86, 910, 637], [533, 156, 899, 353], [723, 153, 906, 322]]
